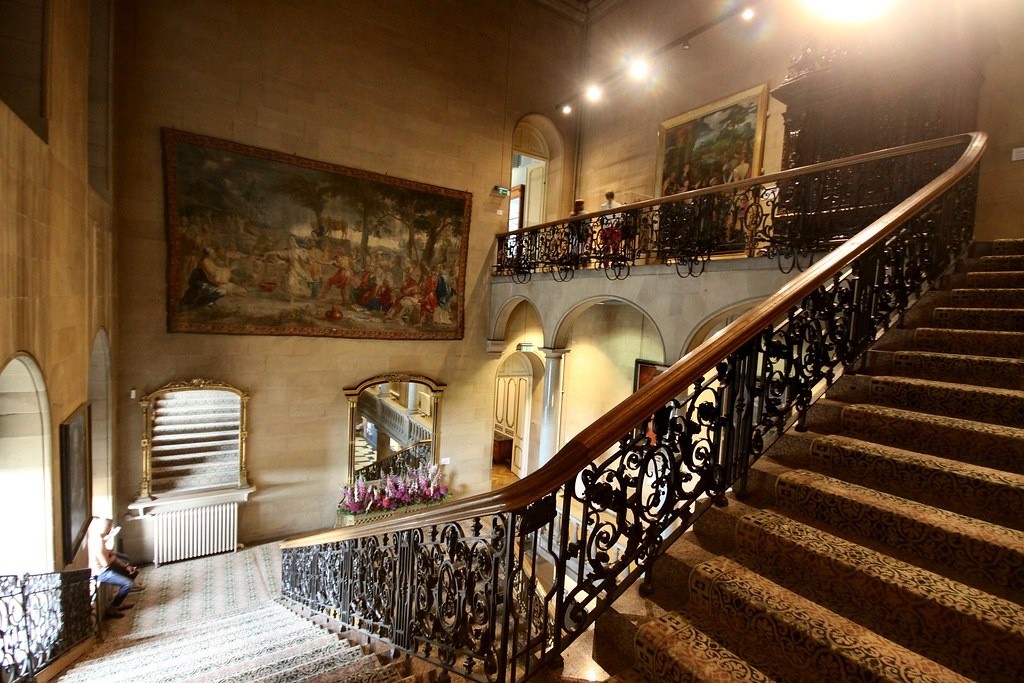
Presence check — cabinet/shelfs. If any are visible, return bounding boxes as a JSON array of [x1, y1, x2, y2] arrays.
[[493, 439, 512, 463]]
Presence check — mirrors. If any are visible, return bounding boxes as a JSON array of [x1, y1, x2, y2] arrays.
[[344, 374, 448, 483]]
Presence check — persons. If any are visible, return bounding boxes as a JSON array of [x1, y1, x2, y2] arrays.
[[89, 516, 146, 618], [568, 199, 591, 270], [600, 192, 623, 270]]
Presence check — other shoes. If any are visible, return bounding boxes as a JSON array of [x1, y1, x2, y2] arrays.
[[117, 603, 134, 609], [127, 583, 146, 595], [104, 608, 126, 618]]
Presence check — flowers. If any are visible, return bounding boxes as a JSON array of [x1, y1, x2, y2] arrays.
[[338, 461, 449, 515]]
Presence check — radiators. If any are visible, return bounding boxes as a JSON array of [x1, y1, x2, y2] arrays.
[[153, 501, 240, 568]]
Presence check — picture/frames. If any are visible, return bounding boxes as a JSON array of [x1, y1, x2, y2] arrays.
[[645, 83, 769, 265], [161, 126, 473, 341]]
